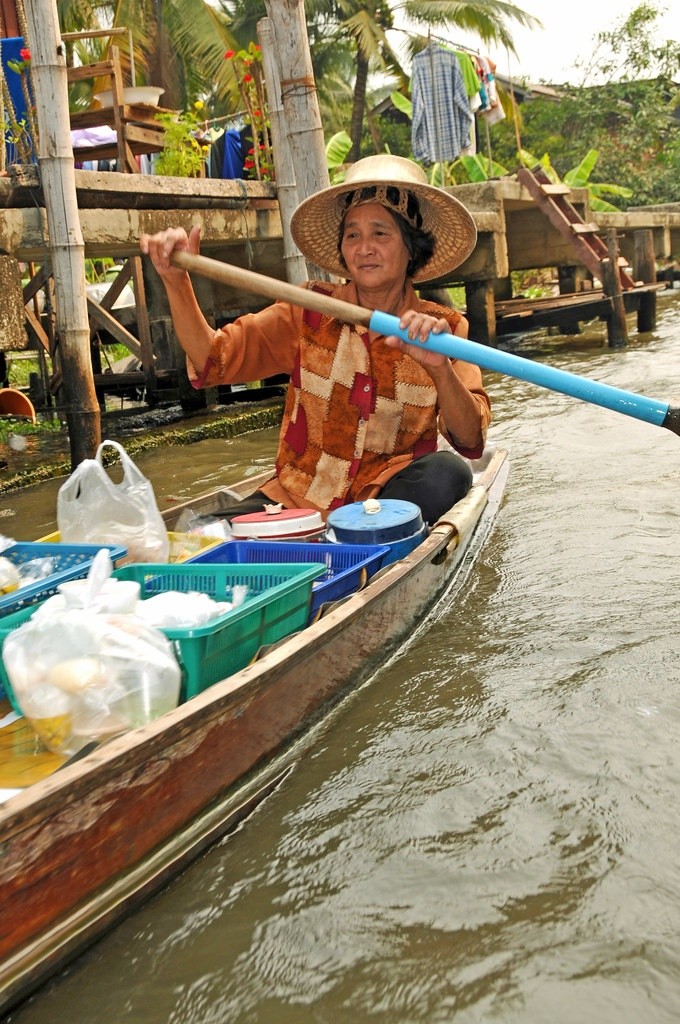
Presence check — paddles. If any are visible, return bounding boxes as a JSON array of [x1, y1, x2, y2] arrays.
[[166, 243, 680, 434]]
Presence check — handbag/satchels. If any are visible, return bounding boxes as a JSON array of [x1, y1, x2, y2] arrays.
[[0, 551, 184, 762], [57, 440, 169, 565]]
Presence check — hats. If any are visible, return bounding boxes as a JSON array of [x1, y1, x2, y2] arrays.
[[287, 154, 480, 284]]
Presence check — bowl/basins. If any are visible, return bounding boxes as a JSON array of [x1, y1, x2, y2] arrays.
[[93, 87, 167, 108]]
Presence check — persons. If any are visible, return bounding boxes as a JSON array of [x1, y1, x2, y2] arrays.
[[140, 155, 491, 530], [654, 254, 679, 288]]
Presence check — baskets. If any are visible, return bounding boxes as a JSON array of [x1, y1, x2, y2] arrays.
[[0, 539, 393, 718]]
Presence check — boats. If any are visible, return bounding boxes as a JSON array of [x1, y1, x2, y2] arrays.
[[1, 446, 516, 1021]]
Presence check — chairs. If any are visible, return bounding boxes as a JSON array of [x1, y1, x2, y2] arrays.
[[68, 53, 182, 173]]
